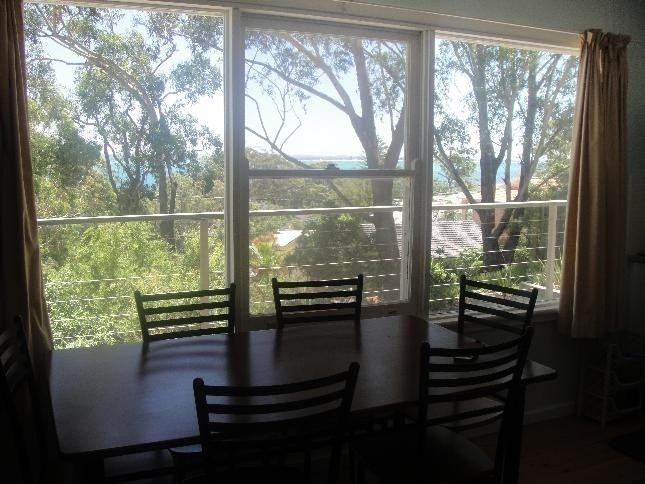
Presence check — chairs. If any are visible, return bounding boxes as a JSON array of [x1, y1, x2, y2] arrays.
[[392, 327, 536, 481], [270, 273, 363, 325], [135, 282, 237, 341], [456, 272, 540, 344], [166, 359, 361, 483]]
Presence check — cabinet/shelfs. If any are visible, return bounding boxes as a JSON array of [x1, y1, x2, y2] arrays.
[[573, 328, 645, 434]]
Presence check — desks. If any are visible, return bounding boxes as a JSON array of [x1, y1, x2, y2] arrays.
[[50, 313, 560, 463]]
